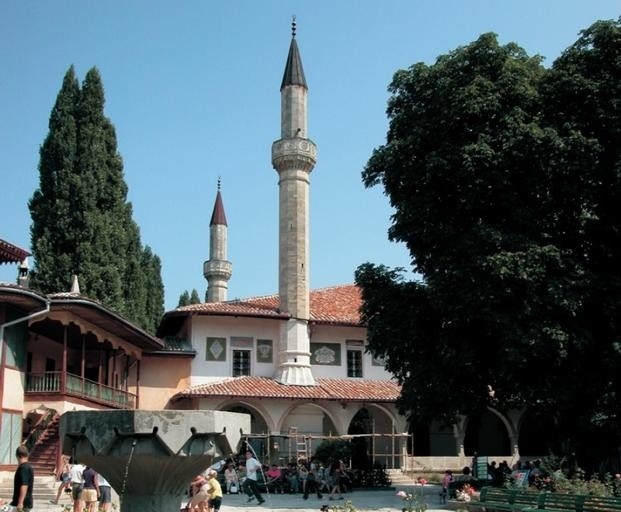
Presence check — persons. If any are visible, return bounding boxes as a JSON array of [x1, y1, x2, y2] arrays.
[[51, 455, 112, 512], [443, 460, 546, 496], [9, 445, 34, 512], [189, 450, 354, 512]]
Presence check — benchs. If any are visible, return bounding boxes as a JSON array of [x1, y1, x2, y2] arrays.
[[466, 484, 620, 512]]
